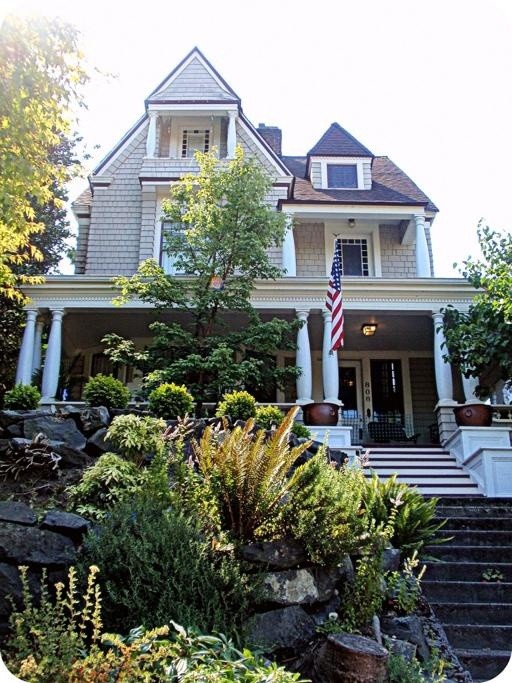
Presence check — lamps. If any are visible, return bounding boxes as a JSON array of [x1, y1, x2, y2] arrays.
[[361, 323, 377, 336], [348, 219, 355, 228]]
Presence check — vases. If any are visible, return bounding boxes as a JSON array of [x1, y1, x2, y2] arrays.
[[301, 402, 339, 426], [453, 403, 493, 426]]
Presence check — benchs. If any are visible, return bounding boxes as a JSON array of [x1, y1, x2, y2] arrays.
[[368, 422, 422, 444]]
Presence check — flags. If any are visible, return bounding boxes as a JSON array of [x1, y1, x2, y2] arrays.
[[324, 239, 346, 353]]
